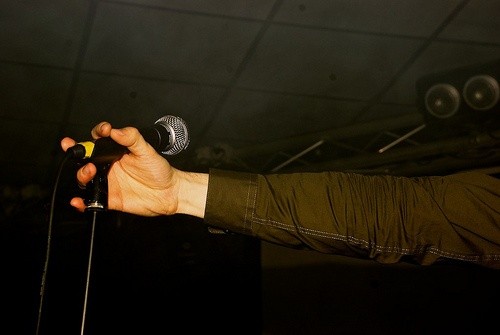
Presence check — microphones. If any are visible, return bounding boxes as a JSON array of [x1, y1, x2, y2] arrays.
[[65, 114, 190, 161]]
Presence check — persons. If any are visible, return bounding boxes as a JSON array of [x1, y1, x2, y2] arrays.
[[59, 122, 500, 269]]
